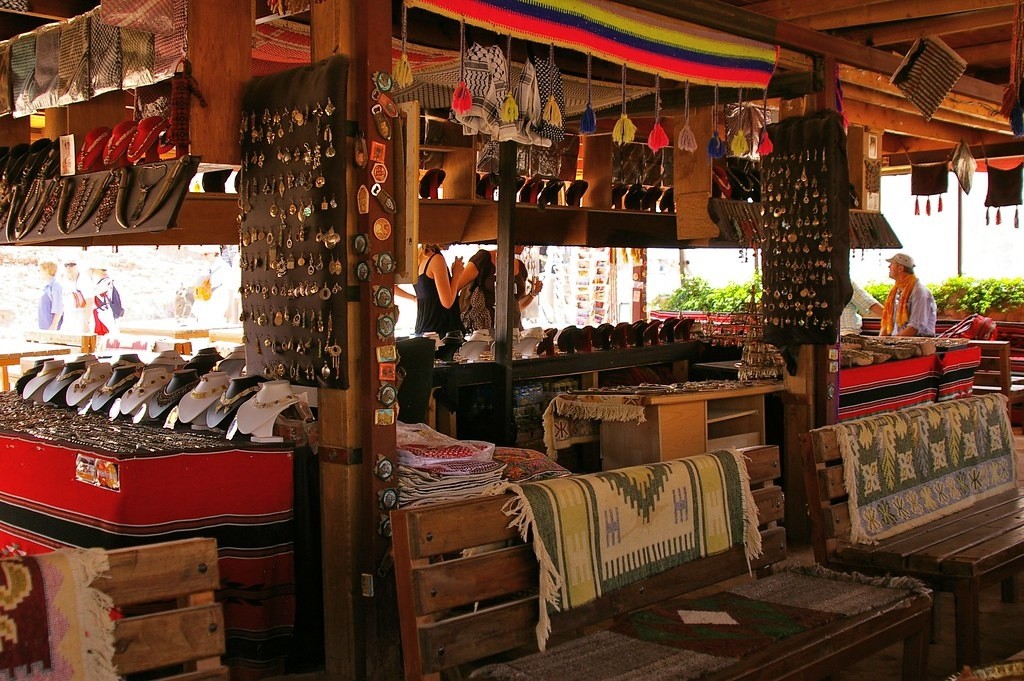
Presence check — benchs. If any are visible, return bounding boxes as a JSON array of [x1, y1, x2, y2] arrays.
[[388, 445, 941, 681], [860, 316, 1024, 425], [798, 392, 1024, 671], [0, 537, 229, 681]]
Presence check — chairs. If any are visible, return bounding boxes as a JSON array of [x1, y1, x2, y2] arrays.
[[964, 339, 1024, 421]]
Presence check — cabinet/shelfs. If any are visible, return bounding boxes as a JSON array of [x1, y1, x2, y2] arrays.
[[419, 115, 681, 249]]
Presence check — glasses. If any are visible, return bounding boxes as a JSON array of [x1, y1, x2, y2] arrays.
[[64, 263, 76, 267]]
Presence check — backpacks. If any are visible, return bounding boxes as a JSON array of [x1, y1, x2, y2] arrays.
[[97, 277, 125, 320]]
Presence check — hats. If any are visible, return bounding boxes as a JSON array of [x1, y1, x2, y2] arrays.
[[885, 253, 914, 269]]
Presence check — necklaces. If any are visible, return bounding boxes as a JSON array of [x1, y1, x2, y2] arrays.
[[849, 192, 860, 207], [0, 115, 167, 239], [517, 169, 641, 197], [712, 167, 761, 194], [433, 312, 690, 365], [0, 345, 305, 454]]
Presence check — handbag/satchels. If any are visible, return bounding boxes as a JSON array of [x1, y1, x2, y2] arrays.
[[460, 285, 493, 333]]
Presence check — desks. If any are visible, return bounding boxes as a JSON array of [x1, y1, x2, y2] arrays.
[[557, 381, 787, 471], [696, 327, 982, 424], [420, 340, 742, 432], [1, 397, 292, 462]]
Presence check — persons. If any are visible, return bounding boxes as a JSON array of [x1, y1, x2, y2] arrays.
[[840, 280, 885, 337], [456, 245, 543, 332], [186, 245, 242, 326], [62, 255, 119, 335], [877, 254, 937, 338], [37, 261, 64, 330], [394, 244, 465, 335]]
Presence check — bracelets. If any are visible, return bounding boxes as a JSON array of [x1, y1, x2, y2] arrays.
[[414, 296, 417, 302], [529, 290, 536, 298]]
